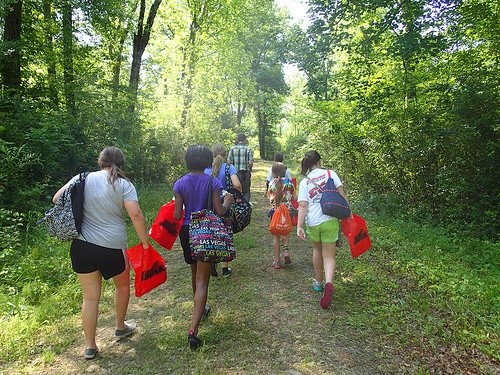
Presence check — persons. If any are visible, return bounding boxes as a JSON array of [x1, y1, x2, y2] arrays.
[[52, 147, 148, 358], [267, 162, 298, 269], [296, 150, 354, 308], [227, 134, 253, 209], [204, 143, 242, 277], [264, 153, 291, 199], [172, 145, 234, 347]]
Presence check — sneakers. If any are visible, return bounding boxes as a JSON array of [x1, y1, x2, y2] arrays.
[[284, 253, 291, 264], [273, 259, 281, 269]]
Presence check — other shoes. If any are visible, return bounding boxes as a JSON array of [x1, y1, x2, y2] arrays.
[[203, 306, 211, 315], [188, 334, 202, 346], [222, 267, 232, 277], [320, 281, 334, 309], [211, 268, 217, 276], [114, 324, 135, 337], [84, 344, 99, 359], [312, 278, 322, 291]]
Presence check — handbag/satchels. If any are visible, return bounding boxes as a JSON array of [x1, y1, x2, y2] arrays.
[[341, 213, 371, 257], [148, 200, 185, 251], [124, 242, 168, 298], [292, 177, 296, 189], [45, 172, 89, 242], [219, 188, 252, 234], [320, 169, 351, 218], [189, 209, 236, 262], [71, 172, 90, 236]]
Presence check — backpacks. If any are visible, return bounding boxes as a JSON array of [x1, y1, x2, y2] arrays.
[[268, 203, 292, 235]]
